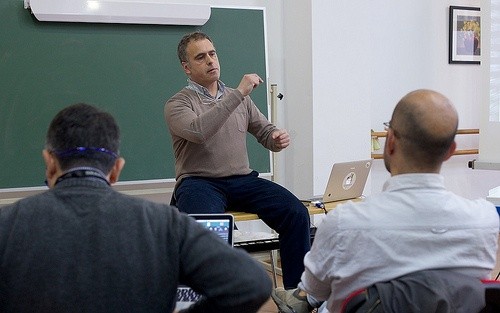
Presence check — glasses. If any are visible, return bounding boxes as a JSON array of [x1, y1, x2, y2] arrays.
[[384, 123, 400, 140]]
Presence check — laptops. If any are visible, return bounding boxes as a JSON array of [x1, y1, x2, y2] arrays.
[[172, 214, 235, 313], [299, 158, 374, 203]]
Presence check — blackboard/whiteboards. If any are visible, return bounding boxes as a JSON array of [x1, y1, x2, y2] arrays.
[[0, 0, 274, 193]]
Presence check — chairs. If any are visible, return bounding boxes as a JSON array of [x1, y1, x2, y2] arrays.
[[341, 280, 500, 313]]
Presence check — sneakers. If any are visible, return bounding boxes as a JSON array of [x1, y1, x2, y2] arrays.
[[271, 289, 314, 313]]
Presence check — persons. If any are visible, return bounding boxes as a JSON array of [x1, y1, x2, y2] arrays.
[[164, 32, 312, 290], [271, 89, 500, 313], [0, 103, 273, 313]]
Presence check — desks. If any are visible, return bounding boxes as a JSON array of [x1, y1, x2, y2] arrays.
[[228, 194, 369, 288]]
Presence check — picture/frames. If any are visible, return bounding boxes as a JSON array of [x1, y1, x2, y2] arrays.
[[449, 5, 481, 64]]
